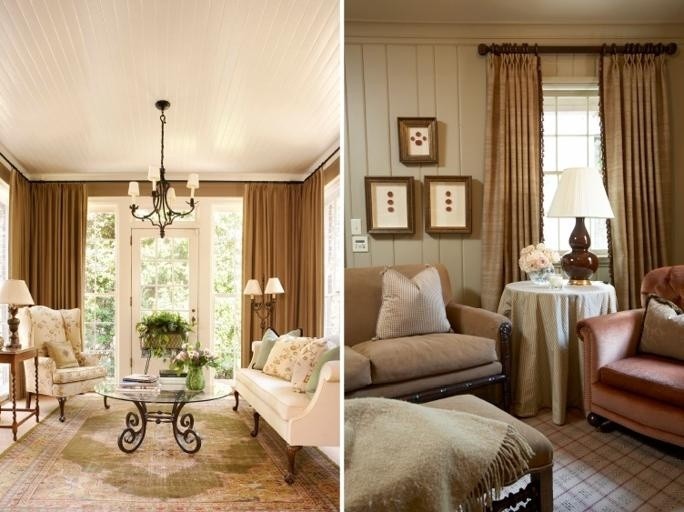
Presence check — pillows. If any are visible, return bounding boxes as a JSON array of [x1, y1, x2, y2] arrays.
[[638, 294, 684, 364], [307, 347, 339, 390], [252, 328, 302, 368], [263, 335, 312, 379], [45, 340, 79, 367], [293, 339, 329, 393], [376, 266, 454, 340]]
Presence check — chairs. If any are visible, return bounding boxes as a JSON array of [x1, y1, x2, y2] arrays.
[[17, 306, 110, 422]]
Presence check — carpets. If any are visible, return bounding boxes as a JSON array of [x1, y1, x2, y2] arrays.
[[0, 395, 340, 512]]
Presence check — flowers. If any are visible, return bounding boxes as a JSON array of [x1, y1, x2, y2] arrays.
[[518, 242, 561, 274], [169, 343, 221, 373]]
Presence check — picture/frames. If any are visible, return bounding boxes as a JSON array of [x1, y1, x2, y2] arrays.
[[396, 115, 440, 166], [364, 174, 416, 240], [423, 175, 474, 237]]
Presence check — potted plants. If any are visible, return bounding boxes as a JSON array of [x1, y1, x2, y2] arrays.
[[137, 310, 196, 357]]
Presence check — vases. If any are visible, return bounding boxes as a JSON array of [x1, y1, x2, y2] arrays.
[[532, 268, 554, 285], [186, 366, 205, 391]]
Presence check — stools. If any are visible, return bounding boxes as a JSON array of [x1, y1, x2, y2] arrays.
[[344, 394, 553, 512]]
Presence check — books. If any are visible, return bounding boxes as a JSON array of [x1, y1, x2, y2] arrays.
[[117, 383, 161, 399], [122, 374, 160, 383]]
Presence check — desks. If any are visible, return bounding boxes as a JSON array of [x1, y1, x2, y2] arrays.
[[0, 348, 39, 440], [496, 281, 619, 426]]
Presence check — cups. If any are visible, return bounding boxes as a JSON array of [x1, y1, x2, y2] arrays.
[[0, 336, 4, 350], [549, 275, 563, 290]]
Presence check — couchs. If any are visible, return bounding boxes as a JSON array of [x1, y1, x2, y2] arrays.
[[575, 266, 684, 453], [232, 334, 340, 483], [345, 264, 512, 413]]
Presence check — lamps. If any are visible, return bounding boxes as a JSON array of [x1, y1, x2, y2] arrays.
[[243, 277, 285, 338], [127, 100, 200, 238], [1, 279, 34, 348], [548, 167, 615, 285]]
